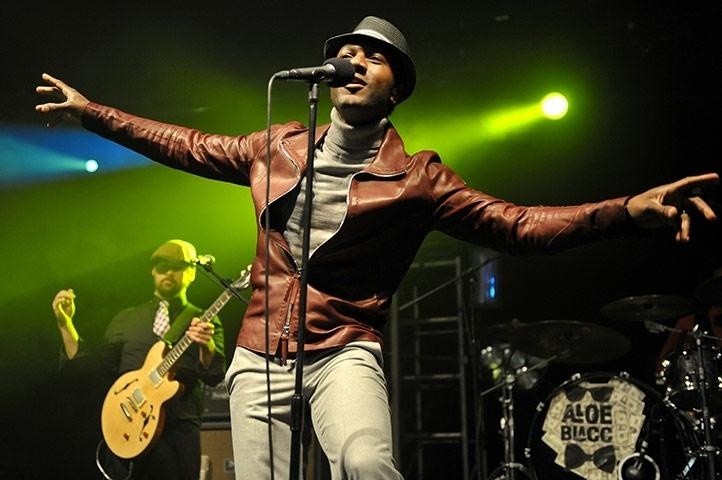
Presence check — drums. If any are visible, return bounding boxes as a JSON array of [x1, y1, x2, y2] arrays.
[[527, 371, 705, 480], [658, 342, 721, 403]]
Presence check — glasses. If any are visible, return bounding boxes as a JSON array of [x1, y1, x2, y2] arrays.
[[154, 261, 187, 275]]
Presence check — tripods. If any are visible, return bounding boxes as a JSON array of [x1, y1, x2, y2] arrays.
[[676, 339, 722, 480]]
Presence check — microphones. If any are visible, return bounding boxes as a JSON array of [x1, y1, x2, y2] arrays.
[[192, 254, 216, 269], [290, 58, 355, 88]]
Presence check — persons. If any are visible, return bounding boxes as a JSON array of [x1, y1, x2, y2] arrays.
[[51, 239, 226, 479], [33, 13, 718, 479], [647, 266, 721, 379]]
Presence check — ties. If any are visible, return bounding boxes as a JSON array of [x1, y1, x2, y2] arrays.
[[152, 300, 173, 350]]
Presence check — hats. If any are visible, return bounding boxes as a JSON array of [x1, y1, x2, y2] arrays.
[[150, 239, 198, 266], [323, 13, 418, 105]]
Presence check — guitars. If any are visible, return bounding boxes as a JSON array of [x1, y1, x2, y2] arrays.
[[101, 263, 252, 460]]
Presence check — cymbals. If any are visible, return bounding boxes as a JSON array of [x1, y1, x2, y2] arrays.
[[514, 321, 632, 364], [601, 295, 698, 319], [462, 322, 518, 355]]
